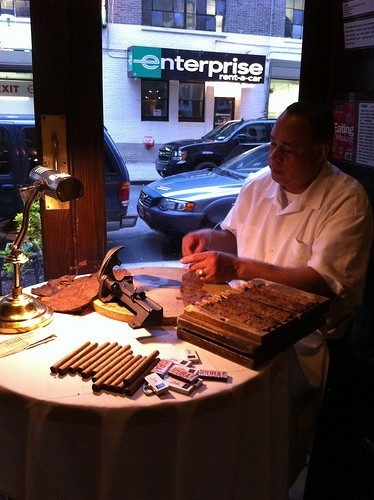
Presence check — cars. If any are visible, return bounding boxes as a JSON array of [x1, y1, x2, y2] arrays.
[[137, 141, 271, 238]]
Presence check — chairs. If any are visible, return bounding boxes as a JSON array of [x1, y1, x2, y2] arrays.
[[304, 162, 374, 500]]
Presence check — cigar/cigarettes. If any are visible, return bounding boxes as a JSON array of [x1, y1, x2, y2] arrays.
[[50, 342, 161, 396]]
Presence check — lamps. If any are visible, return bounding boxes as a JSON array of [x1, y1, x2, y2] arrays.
[[0, 165, 85, 334]]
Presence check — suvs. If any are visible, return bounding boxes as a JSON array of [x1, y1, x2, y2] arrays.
[[0, 114, 138, 233], [155, 116, 278, 178]]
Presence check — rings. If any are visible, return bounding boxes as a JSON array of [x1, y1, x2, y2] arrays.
[[199, 269, 202, 276]]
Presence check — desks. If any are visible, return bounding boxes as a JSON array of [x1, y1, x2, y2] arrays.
[[0, 262, 329, 500]]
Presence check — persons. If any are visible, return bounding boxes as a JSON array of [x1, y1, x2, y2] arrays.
[[182, 103, 374, 354]]
